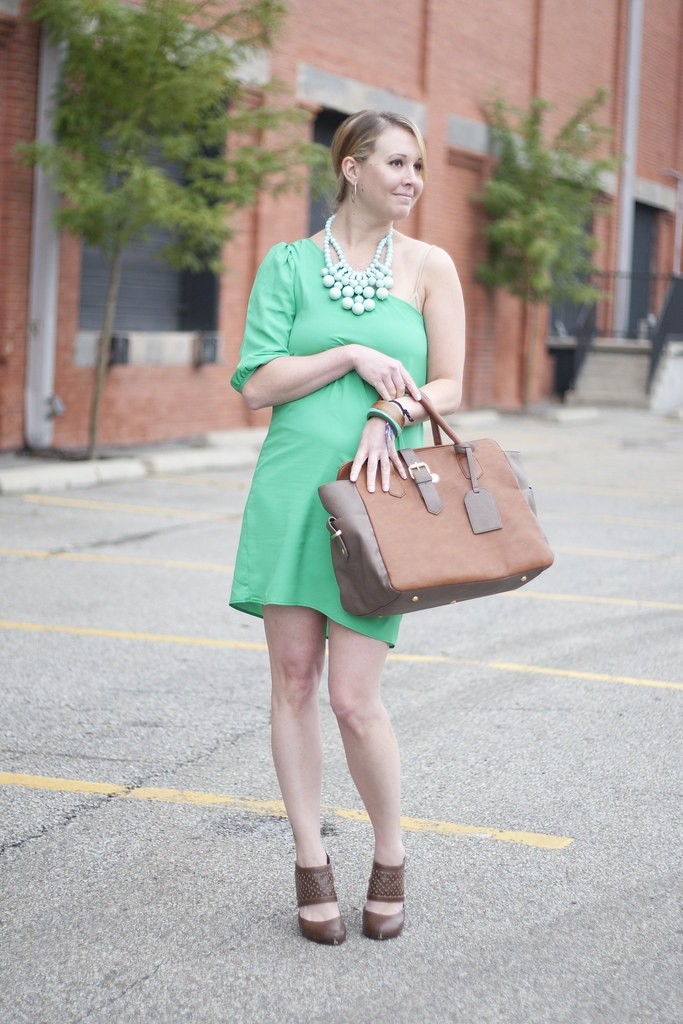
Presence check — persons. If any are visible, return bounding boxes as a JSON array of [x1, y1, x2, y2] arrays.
[[232, 111, 466, 945]]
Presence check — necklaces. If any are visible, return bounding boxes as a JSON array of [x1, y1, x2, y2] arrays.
[[319, 211, 396, 317]]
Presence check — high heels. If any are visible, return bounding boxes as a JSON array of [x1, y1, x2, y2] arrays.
[[295, 854, 346, 945], [363, 854, 406, 939]]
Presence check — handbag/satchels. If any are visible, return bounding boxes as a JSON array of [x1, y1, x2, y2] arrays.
[[317, 387, 554, 615]]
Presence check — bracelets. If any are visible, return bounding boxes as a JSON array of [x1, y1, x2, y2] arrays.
[[383, 399, 415, 423], [365, 410, 404, 435]]
